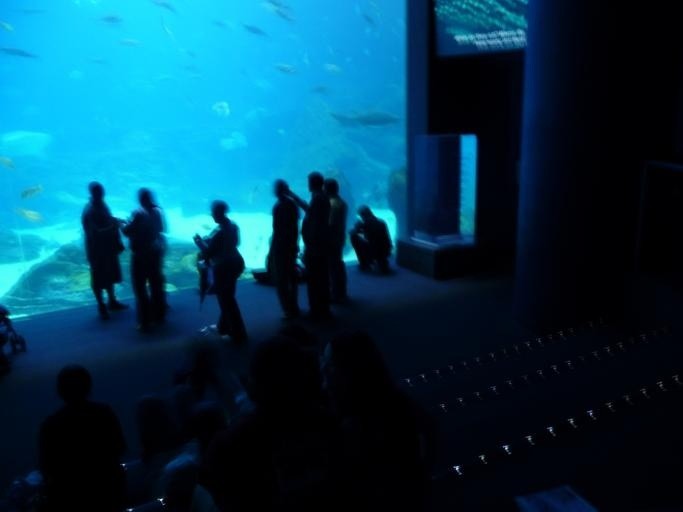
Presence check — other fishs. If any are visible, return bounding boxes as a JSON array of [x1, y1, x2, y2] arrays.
[[21, 209, 41, 221], [0, 0, 407, 94], [20, 184, 41, 198], [352, 111, 399, 127], [2, 156, 16, 168]]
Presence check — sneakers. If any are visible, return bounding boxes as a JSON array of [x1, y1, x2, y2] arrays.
[[97, 303, 109, 320], [107, 300, 129, 311]]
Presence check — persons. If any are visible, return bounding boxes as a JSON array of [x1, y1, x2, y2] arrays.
[[84, 183, 128, 320], [349, 208, 390, 270], [286, 173, 330, 319], [123, 186, 166, 331], [205, 201, 247, 344], [322, 180, 347, 303], [269, 182, 299, 318]]
